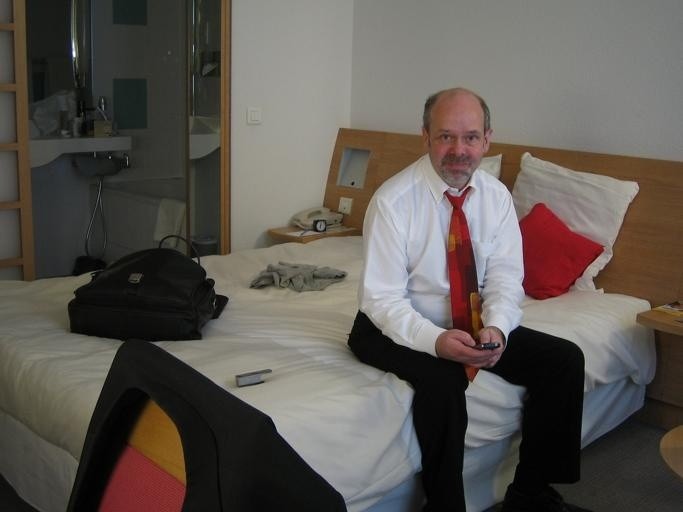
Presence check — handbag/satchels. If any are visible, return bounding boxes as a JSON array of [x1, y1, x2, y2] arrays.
[[67, 247, 216, 341]]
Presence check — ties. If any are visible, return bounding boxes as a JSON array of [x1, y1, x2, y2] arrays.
[[444, 186, 484, 382]]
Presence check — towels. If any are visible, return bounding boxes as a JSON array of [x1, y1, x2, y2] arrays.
[[152, 197, 186, 249]]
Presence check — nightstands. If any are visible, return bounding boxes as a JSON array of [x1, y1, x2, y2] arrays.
[[635, 298, 683, 477], [268, 220, 361, 244]]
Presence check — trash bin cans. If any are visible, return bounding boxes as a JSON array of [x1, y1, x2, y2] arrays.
[[191, 236, 218, 257]]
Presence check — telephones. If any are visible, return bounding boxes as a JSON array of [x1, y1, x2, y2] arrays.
[[292, 207, 343, 229]]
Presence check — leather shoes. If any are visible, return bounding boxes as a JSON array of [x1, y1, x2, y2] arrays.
[[504, 483, 589, 511]]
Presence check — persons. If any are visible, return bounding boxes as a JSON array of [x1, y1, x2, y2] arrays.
[[344, 87, 596, 511]]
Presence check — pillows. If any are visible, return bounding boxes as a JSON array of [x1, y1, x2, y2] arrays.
[[476, 152, 502, 179], [519, 204, 605, 299], [512, 151, 640, 294]]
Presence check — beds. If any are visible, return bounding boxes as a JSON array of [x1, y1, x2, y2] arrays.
[[1, 128, 683, 512]]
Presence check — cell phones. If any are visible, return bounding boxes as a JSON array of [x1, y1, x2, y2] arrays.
[[474, 341, 501, 352]]
